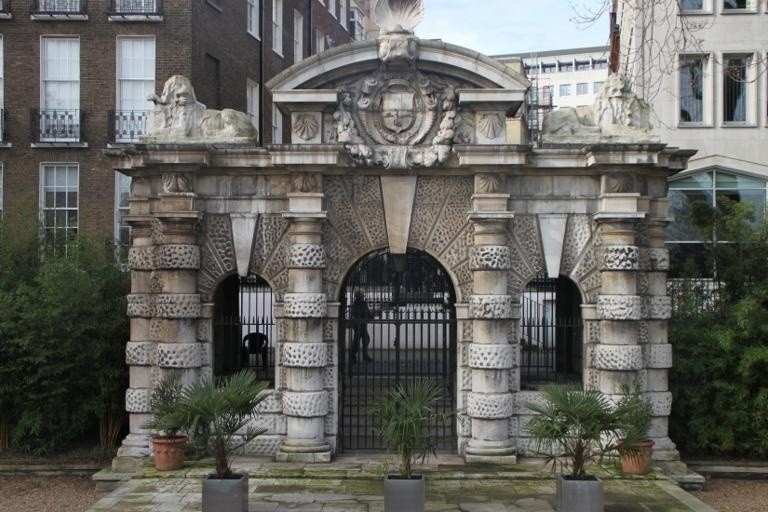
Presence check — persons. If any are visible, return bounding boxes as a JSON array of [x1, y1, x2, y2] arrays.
[[351, 290, 374, 363]]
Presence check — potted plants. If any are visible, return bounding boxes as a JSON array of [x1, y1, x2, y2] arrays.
[[616, 374, 656, 475], [367, 372, 466, 511], [178, 365, 274, 511], [521, 377, 647, 510], [145, 368, 195, 471]]
